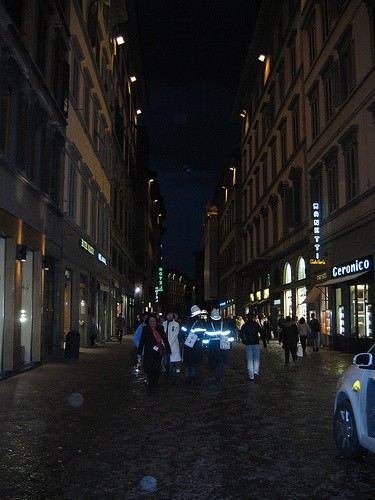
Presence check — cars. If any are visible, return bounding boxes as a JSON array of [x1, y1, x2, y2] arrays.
[[329, 344, 375, 460]]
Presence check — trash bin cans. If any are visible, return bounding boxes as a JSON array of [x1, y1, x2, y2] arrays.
[[64, 329, 81, 359]]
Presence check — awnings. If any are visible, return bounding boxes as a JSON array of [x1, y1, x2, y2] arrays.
[[315, 269, 372, 300], [243, 300, 267, 307], [302, 284, 326, 303]]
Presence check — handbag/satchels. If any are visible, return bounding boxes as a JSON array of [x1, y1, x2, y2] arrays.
[[158, 344, 166, 356]]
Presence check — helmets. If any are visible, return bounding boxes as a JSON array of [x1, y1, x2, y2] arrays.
[[191, 305, 201, 316], [210, 309, 221, 320]]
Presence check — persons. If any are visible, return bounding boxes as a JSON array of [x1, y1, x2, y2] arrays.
[[230, 312, 277, 347], [278, 315, 310, 365], [240, 316, 265, 381], [308, 313, 321, 352], [133, 305, 234, 392], [114, 313, 126, 344], [88, 316, 98, 346]]
